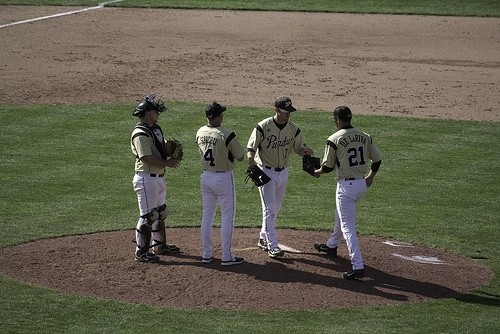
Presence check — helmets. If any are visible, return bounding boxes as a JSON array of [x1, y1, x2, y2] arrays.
[[133, 101, 157, 116]]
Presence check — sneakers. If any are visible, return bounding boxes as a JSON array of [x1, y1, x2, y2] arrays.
[[221, 257, 244, 265], [203, 257, 214, 262], [268, 247, 284, 258], [343, 269, 365, 280], [152, 245, 179, 254], [258, 239, 268, 251], [314, 242, 337, 257], [135, 252, 159, 262]]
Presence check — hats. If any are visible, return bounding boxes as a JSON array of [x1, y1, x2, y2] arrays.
[[205, 101, 226, 118], [275, 96, 297, 113], [330, 106, 352, 121]]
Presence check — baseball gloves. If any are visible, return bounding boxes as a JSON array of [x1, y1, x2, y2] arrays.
[[248, 165, 271, 186], [302, 154, 321, 178], [165, 139, 183, 160]]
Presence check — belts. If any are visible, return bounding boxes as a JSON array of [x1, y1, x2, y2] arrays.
[[345, 177, 364, 181], [204, 169, 225, 173], [137, 172, 164, 177], [265, 166, 287, 172]]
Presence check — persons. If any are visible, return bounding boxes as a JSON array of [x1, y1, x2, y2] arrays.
[[195, 102, 244, 266], [130, 93, 181, 262], [244, 96, 314, 260], [312, 106, 381, 279]]
